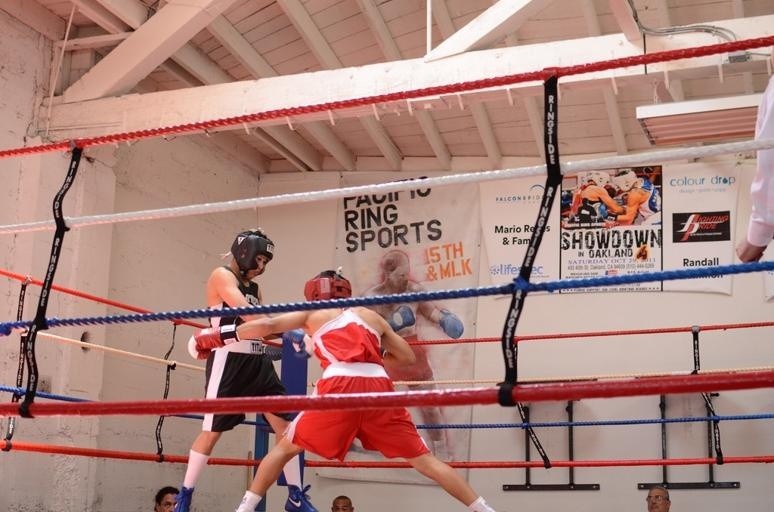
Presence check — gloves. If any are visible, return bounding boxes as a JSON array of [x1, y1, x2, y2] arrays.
[[188, 324, 239, 360], [282, 328, 314, 360], [599, 204, 609, 217], [439, 309, 464, 339], [386, 306, 415, 331]]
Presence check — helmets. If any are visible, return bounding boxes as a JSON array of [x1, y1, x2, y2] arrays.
[[588, 170, 639, 197], [304, 271, 351, 302], [231, 231, 274, 270]]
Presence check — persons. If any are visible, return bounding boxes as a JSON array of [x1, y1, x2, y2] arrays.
[[564, 169, 661, 226], [645, 485, 672, 512], [734, 46, 774, 264], [154, 486, 180, 512], [186, 266, 497, 512], [360, 249, 463, 463], [331, 495, 354, 512], [172, 227, 321, 512]]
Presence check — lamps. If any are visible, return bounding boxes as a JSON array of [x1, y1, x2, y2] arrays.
[[635, 79, 764, 149]]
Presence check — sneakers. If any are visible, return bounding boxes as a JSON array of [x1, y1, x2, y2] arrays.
[[174, 487, 195, 512], [284, 484, 318, 512]]
